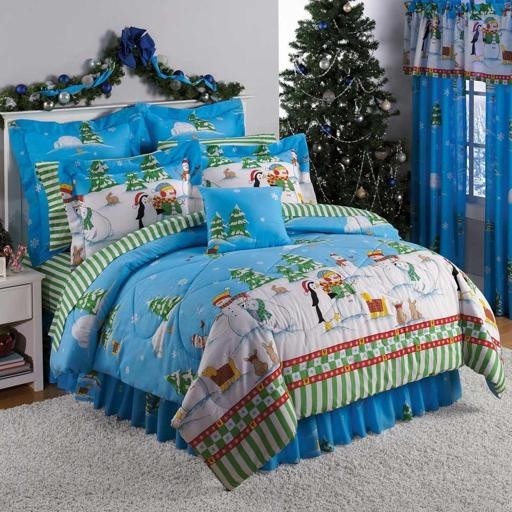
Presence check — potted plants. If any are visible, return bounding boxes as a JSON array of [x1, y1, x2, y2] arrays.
[[0, 219, 12, 280]]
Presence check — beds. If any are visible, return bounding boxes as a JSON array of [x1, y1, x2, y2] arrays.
[[1, 95, 502, 471]]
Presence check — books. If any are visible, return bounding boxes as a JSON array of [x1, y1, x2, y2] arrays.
[[0, 352, 32, 379]]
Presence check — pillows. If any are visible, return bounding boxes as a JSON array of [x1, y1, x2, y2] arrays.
[[8, 102, 150, 268], [156, 133, 277, 151], [141, 98, 246, 151], [198, 185, 294, 254], [178, 133, 318, 204], [58, 139, 203, 271], [35, 160, 72, 252]]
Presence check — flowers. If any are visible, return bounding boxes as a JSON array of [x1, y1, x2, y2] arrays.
[[118, 27, 156, 69]]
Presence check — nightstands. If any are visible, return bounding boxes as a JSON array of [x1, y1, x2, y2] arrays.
[[0, 262, 46, 392]]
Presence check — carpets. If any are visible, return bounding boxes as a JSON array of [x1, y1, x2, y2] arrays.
[[1, 345, 512, 512]]
